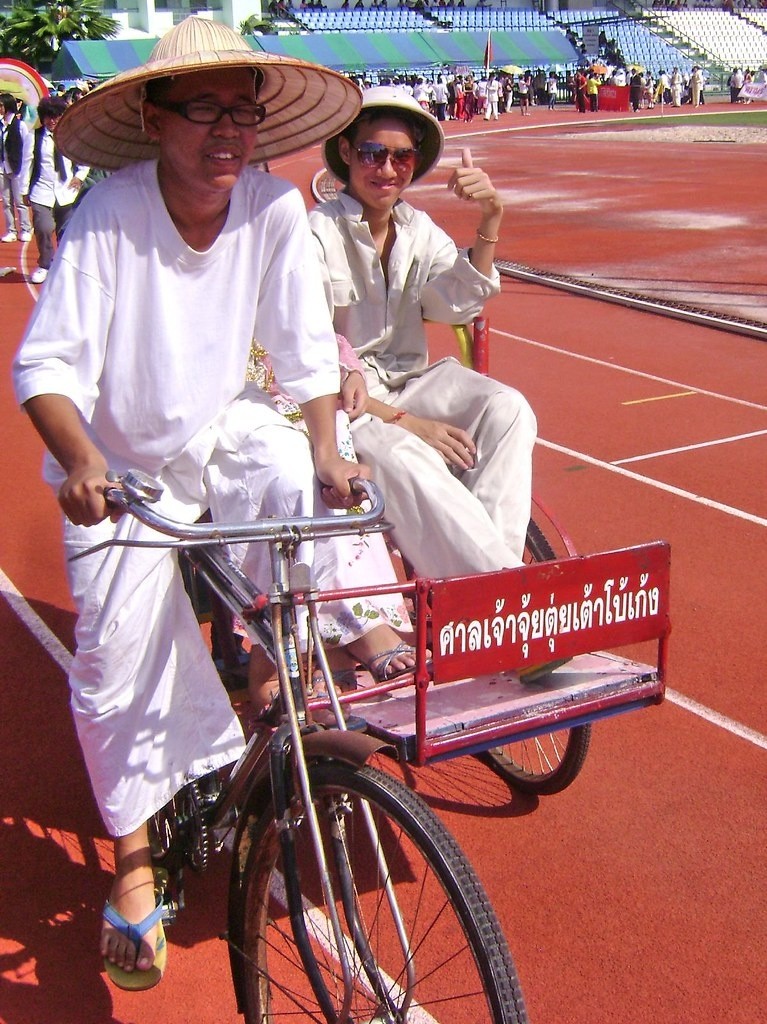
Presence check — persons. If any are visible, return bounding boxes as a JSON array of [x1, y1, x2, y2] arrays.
[[10, 17, 373, 991], [246, 331, 432, 695], [0, 79, 118, 284], [565, 25, 626, 66], [268, 0, 767, 18], [727, 65, 767, 104], [308, 86, 574, 685], [348, 66, 709, 123]]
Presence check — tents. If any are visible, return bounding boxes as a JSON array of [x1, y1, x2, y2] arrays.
[[52, 38, 161, 85], [244, 29, 580, 71]]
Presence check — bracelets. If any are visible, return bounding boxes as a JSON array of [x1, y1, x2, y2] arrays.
[[384, 410, 407, 424], [476, 228, 498, 243]]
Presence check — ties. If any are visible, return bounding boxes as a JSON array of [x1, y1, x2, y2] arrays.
[[50, 135, 67, 181], [0, 124, 8, 162]]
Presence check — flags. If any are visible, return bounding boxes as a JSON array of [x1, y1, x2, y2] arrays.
[[484, 33, 493, 68]]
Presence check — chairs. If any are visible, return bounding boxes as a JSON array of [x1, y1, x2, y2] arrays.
[[290, 7, 767, 82]]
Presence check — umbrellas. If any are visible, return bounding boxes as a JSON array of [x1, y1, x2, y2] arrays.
[[627, 64, 646, 73], [546, 64, 565, 72], [588, 66, 608, 74], [501, 65, 523, 74]]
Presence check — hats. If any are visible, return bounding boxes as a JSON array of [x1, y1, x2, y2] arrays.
[[321, 86, 444, 187], [54, 17, 363, 174]]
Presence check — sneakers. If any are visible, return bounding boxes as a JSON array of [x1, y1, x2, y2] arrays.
[[20, 231, 31, 241], [31, 267, 48, 283], [2, 233, 18, 243]]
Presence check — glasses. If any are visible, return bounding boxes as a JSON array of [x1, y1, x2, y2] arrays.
[[351, 142, 418, 172], [145, 96, 266, 126]]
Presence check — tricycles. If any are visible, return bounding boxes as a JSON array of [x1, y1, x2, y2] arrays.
[[57, 291, 678, 1024]]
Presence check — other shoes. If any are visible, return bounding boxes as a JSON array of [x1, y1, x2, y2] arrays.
[[515, 657, 575, 684]]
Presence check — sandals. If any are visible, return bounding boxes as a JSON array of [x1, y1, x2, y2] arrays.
[[311, 663, 356, 694], [340, 639, 435, 682]]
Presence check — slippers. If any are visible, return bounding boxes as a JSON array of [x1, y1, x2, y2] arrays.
[[316, 693, 368, 733], [100, 867, 169, 991]]
